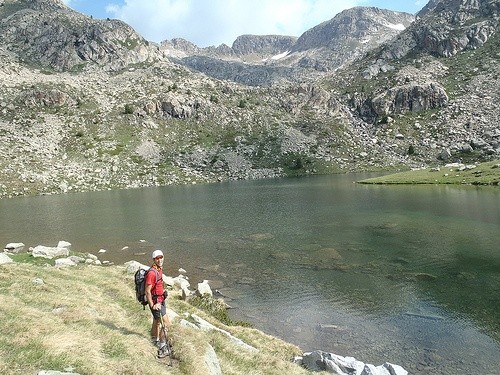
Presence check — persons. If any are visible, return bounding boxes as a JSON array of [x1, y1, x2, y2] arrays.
[[145, 249, 175, 359]]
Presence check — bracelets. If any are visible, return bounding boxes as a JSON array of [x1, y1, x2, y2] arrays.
[[151, 304, 154, 309]]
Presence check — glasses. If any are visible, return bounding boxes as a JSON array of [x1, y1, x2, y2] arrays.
[[154, 257, 163, 259]]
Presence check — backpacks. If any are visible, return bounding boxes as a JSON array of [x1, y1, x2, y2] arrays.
[[134, 266, 159, 309]]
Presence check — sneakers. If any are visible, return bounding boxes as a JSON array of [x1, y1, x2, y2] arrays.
[[151, 337, 161, 345], [158, 344, 173, 357]]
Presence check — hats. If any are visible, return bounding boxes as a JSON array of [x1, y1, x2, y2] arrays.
[[151, 249, 164, 258]]
